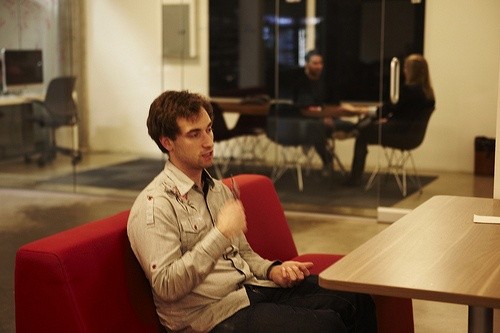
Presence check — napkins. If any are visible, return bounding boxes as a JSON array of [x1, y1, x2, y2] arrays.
[[473, 214, 500, 224]]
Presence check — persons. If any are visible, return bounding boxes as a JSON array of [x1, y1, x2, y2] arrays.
[[127, 89, 376, 331], [347, 54, 435, 193], [295, 50, 367, 189]]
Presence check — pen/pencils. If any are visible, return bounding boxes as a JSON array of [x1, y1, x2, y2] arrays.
[[230, 174, 238, 201]]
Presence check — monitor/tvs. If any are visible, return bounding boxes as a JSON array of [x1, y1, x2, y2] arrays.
[[1, 48, 45, 93]]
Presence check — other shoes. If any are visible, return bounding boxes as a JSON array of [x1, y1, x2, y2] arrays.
[[339, 179, 360, 187]]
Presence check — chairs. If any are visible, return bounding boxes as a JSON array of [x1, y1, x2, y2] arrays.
[[366, 107, 435, 197], [261, 100, 322, 191], [24, 76, 81, 166], [210, 101, 262, 180]]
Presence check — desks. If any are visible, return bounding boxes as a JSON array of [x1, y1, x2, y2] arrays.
[[0, 92, 77, 158], [318, 195, 500, 333]]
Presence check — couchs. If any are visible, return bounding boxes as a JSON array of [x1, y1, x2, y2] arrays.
[[14, 174, 344, 333]]
